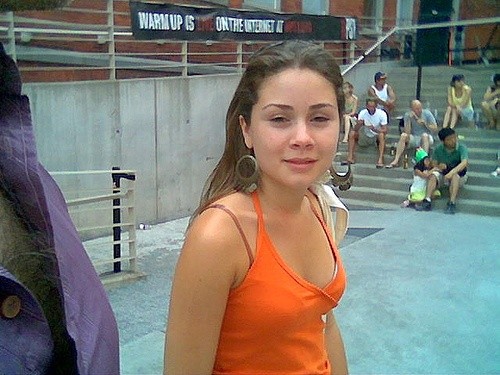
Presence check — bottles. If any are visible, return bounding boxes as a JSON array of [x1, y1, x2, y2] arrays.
[[403, 154, 409, 169], [390, 145, 395, 156]]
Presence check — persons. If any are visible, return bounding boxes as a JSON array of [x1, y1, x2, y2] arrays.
[[443, 74, 474, 129], [400, 148, 446, 208], [341, 97, 388, 168], [414, 128, 470, 214], [341, 82, 358, 142], [385, 99, 437, 168], [164, 39, 350, 375], [481, 73, 500, 131], [0, 0, 119, 375], [368, 72, 396, 125]]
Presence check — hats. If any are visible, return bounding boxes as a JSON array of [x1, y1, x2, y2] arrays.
[[413, 147, 428, 163], [378, 75, 388, 79]]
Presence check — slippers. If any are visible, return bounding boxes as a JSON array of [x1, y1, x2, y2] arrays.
[[340, 160, 355, 166], [385, 164, 400, 169], [376, 163, 383, 168]]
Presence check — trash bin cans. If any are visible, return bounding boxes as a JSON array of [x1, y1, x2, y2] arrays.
[[413, 14, 451, 64]]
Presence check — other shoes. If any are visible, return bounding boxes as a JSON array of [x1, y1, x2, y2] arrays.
[[445, 200, 456, 214], [417, 199, 431, 211]]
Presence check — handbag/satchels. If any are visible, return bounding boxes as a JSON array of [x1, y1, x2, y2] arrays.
[[443, 160, 468, 176]]
